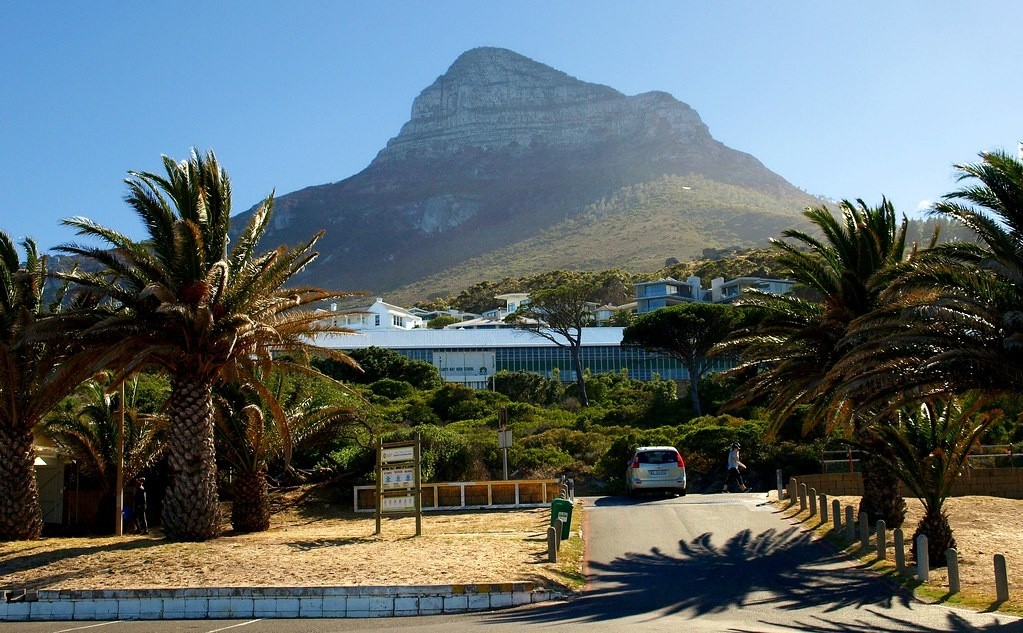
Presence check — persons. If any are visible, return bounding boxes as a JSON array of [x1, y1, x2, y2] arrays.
[[722, 442, 752, 493], [134, 476, 149, 535]]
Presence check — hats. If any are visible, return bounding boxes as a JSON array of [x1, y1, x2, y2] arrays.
[[733, 442, 741, 449]]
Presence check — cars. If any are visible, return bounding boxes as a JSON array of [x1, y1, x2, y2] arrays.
[[627, 445, 687, 495]]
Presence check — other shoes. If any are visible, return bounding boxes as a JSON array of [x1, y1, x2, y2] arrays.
[[742, 488, 752, 493], [722, 489, 729, 493]]
